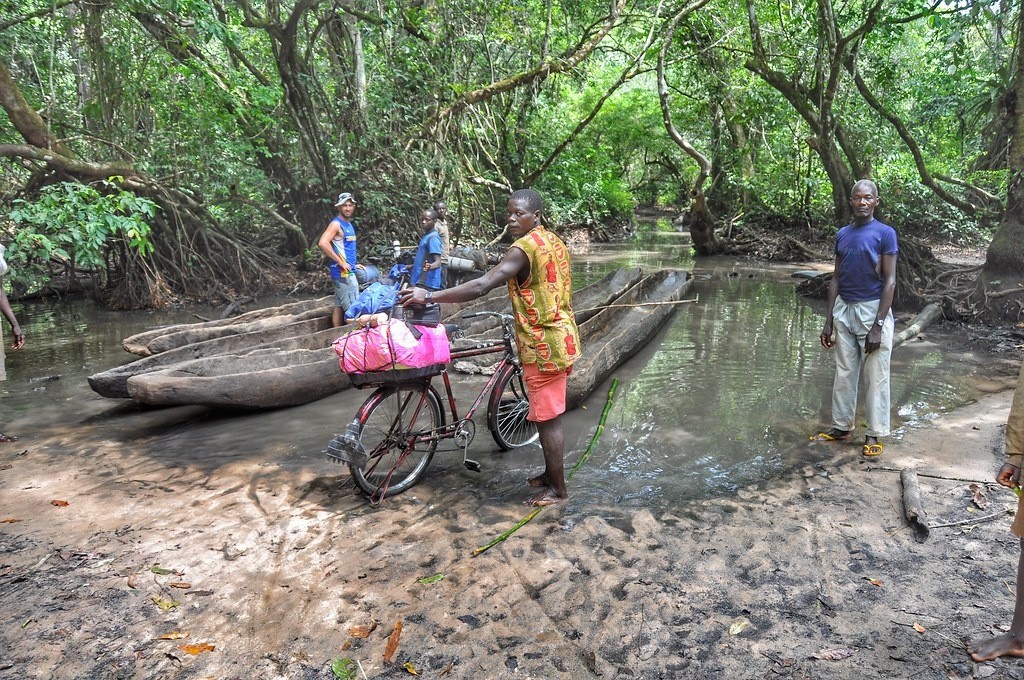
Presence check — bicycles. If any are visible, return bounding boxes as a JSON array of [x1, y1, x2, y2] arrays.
[[331, 311, 546, 504]]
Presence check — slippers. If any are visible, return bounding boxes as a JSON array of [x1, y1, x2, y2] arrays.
[[809, 433, 851, 442], [865, 443, 883, 456]]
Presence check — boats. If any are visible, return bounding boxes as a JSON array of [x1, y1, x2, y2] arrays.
[[121, 295, 333, 358], [488, 266, 697, 427], [125, 278, 512, 404], [451, 264, 644, 379], [87, 311, 347, 399]]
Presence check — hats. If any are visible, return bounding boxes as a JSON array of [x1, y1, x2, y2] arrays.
[[335, 193, 357, 209]]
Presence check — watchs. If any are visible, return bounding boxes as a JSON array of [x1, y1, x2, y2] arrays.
[[873, 318, 885, 327], [425, 292, 433, 304]]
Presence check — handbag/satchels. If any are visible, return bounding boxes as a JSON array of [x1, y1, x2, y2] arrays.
[[331, 282, 450, 376]]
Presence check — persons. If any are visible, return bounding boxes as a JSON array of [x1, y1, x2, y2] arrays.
[[398, 189, 581, 507], [318, 192, 360, 327], [403, 201, 449, 291], [809, 179, 897, 461], [966, 354, 1024, 662]]
